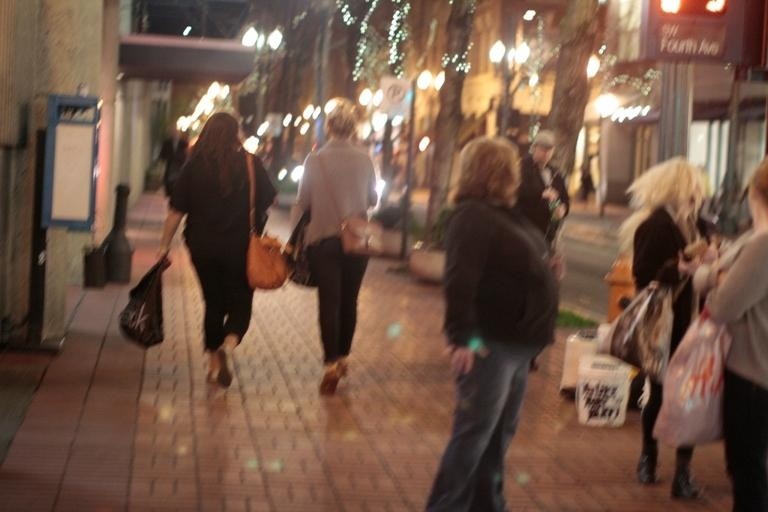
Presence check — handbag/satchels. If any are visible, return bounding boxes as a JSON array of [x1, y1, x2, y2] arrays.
[[342, 217, 371, 254], [247, 153, 316, 289], [596, 280, 730, 447], [119, 256, 172, 349]]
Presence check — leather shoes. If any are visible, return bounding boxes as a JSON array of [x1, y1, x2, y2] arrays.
[[319, 358, 348, 396]]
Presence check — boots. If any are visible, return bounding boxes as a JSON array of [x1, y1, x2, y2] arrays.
[[671, 465, 699, 500], [637, 446, 657, 484]]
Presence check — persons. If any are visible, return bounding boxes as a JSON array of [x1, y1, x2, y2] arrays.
[[292, 100, 379, 394], [625, 156, 722, 497], [706, 156, 768, 512], [516, 130, 570, 366], [580, 166, 595, 208], [158, 110, 320, 196], [154, 113, 280, 384], [426, 136, 563, 511]]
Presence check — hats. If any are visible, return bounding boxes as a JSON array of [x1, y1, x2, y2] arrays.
[[535, 130, 555, 149]]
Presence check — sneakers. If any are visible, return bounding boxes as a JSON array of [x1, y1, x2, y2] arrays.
[[206, 344, 232, 389]]
